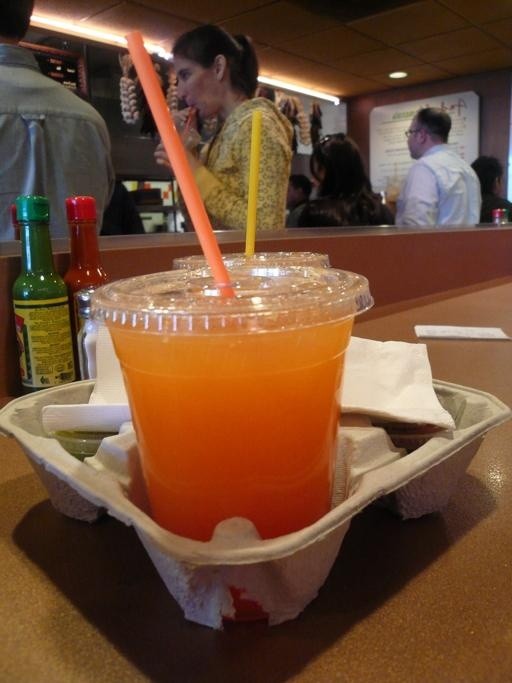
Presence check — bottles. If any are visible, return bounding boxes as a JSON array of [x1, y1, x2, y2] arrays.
[[12, 196, 80, 396], [63, 195, 117, 340]]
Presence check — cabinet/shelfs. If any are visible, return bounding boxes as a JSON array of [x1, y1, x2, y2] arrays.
[[117, 175, 183, 233]]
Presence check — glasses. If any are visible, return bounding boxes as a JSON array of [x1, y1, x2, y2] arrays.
[[406, 128, 428, 139]]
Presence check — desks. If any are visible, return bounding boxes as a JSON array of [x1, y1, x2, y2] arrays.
[[0, 225, 512, 683]]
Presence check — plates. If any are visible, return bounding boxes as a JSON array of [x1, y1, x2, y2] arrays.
[[1, 376, 512, 630]]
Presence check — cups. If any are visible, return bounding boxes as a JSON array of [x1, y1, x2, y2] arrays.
[[171, 250, 333, 270], [89, 269, 378, 542], [173, 115, 207, 156]]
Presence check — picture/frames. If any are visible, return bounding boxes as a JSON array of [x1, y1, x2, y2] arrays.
[[369, 91, 480, 204]]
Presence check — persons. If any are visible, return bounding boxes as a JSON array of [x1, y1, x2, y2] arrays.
[[287, 174, 314, 227], [154, 22, 294, 231], [470, 156, 512, 223], [0, 1, 114, 240], [286, 133, 395, 227], [395, 107, 482, 226]]
